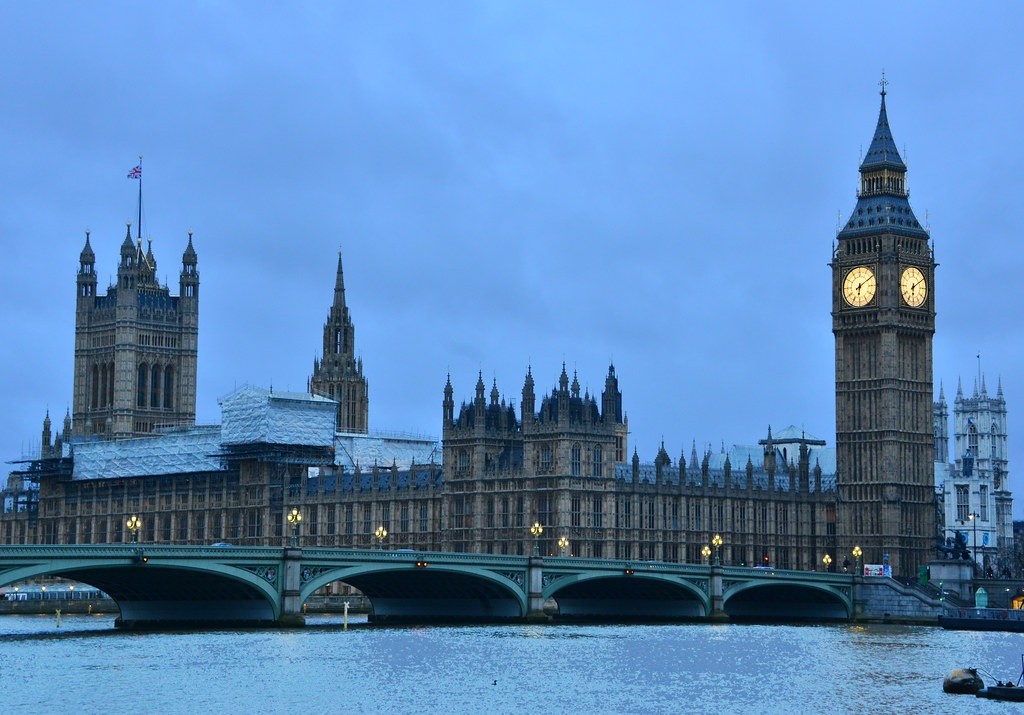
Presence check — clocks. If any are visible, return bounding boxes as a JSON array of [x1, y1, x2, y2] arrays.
[[901, 267, 928, 307], [843, 265, 877, 306]]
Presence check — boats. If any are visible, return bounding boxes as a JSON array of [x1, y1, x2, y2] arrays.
[[978, 652, 1024, 702]]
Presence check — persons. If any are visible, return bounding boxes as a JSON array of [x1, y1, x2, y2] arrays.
[[843, 556, 850, 574], [987, 566, 994, 579], [1000, 567, 1012, 579]]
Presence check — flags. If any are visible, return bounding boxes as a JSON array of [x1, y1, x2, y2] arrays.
[[127, 164, 142, 179]]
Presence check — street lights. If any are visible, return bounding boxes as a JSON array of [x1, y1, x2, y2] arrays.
[[15, 586, 18, 600], [375, 526, 387, 551], [69, 585, 74, 599], [823, 555, 832, 572], [531, 521, 543, 556], [126, 514, 143, 544], [968, 510, 977, 578], [853, 545, 863, 576], [558, 536, 569, 556], [702, 546, 711, 564], [42, 585, 45, 600], [287, 506, 302, 547], [1005, 587, 1010, 609], [712, 533, 723, 565]]
